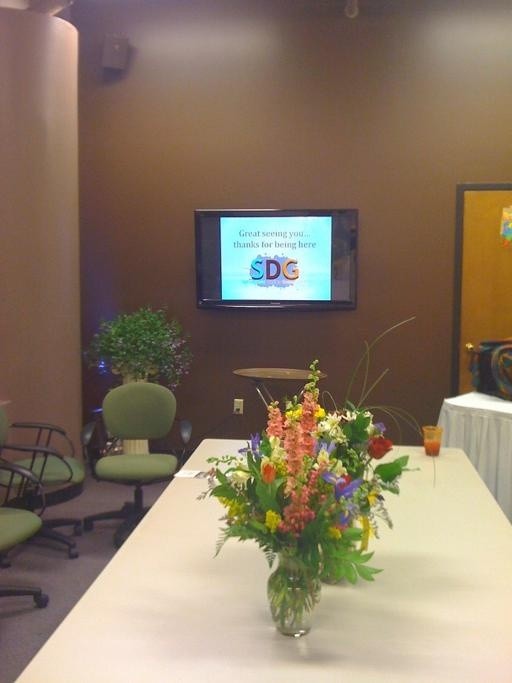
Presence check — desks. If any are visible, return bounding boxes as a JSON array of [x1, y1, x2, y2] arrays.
[[231, 367, 327, 414]]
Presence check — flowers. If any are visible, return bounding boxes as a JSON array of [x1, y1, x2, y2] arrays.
[[84, 307, 193, 391], [195, 363, 413, 628]]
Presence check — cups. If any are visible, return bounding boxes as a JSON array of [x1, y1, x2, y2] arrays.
[[423, 424, 444, 457]]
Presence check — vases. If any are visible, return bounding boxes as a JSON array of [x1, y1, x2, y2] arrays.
[[266, 553, 321, 637], [120, 367, 150, 454]]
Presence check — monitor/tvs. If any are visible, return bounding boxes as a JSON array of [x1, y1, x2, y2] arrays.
[[194, 208, 359, 312]]
